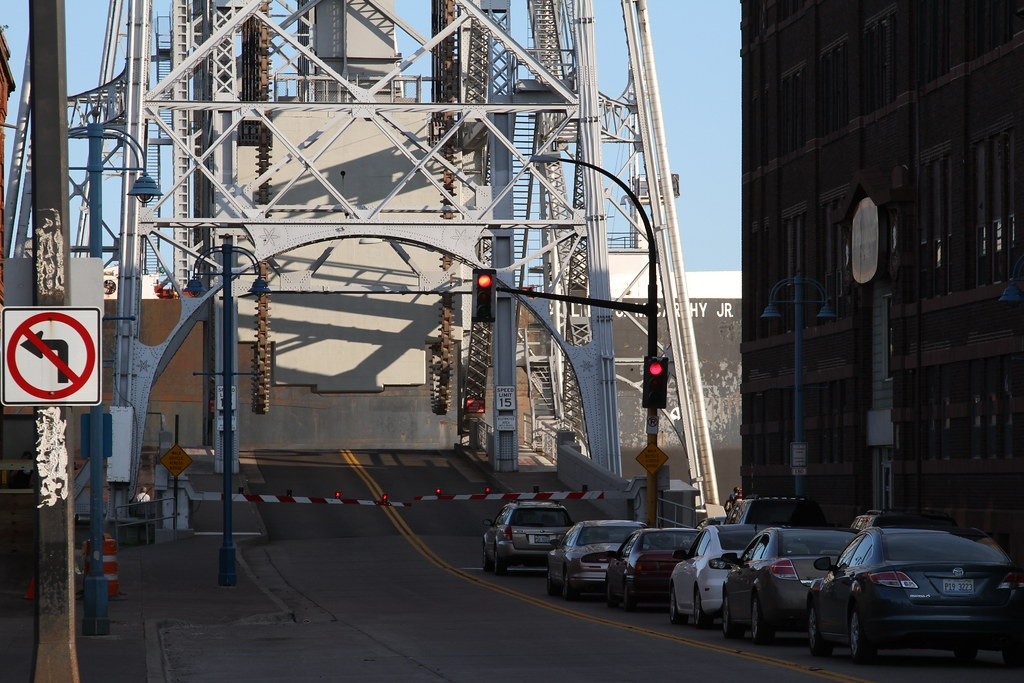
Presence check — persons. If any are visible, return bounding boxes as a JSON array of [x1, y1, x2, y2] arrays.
[[724, 487, 743, 517], [136, 487, 150, 502]]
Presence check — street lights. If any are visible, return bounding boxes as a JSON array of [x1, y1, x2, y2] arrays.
[[74, 109, 165, 636], [760, 272, 839, 498], [998, 254, 1024, 309], [182, 235, 273, 588]]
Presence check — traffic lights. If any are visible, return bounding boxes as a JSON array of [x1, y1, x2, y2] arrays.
[[642, 355, 668, 409], [472, 268, 497, 323]]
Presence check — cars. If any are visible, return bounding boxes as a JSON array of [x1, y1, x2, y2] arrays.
[[481, 496, 1024, 665]]
